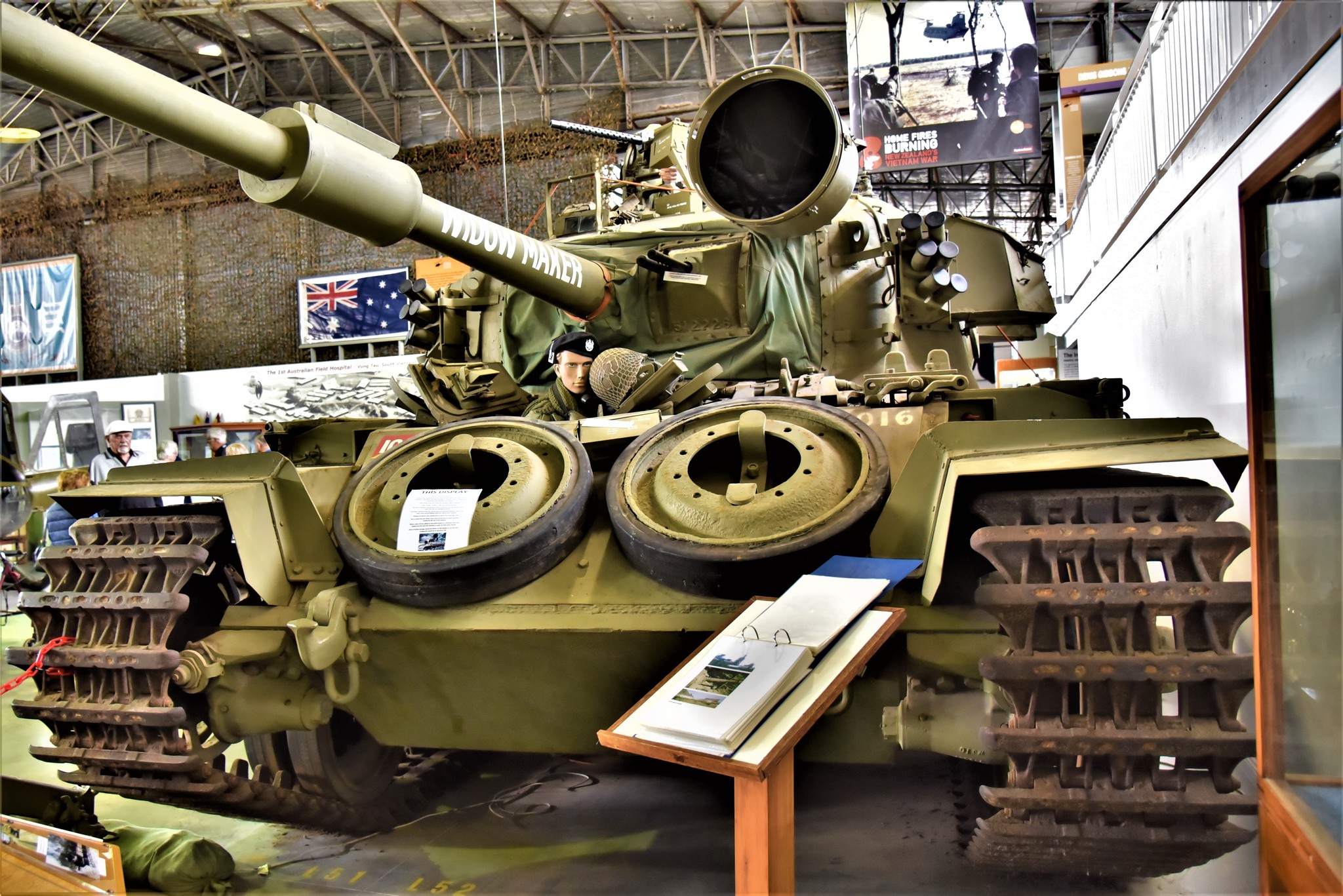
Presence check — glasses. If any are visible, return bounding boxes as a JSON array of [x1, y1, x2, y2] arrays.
[[207, 437, 216, 442]]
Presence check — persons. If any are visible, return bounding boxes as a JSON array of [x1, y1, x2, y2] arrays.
[[45, 467, 99, 547], [226, 442, 249, 456], [968, 43, 1042, 119], [88, 421, 158, 510], [206, 427, 230, 457], [254, 433, 272, 453], [520, 331, 600, 422], [151, 440, 178, 465], [622, 124, 690, 223], [854, 65, 906, 134]]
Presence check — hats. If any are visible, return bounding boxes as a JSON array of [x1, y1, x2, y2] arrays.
[[545, 331, 599, 360], [105, 420, 133, 437]]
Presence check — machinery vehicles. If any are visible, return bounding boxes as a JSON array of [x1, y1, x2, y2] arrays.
[[2, 0, 1258, 896]]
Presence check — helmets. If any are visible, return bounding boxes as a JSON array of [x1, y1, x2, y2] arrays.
[[591, 348, 649, 409]]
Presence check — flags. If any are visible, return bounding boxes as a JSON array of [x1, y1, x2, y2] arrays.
[[301, 271, 409, 339]]
